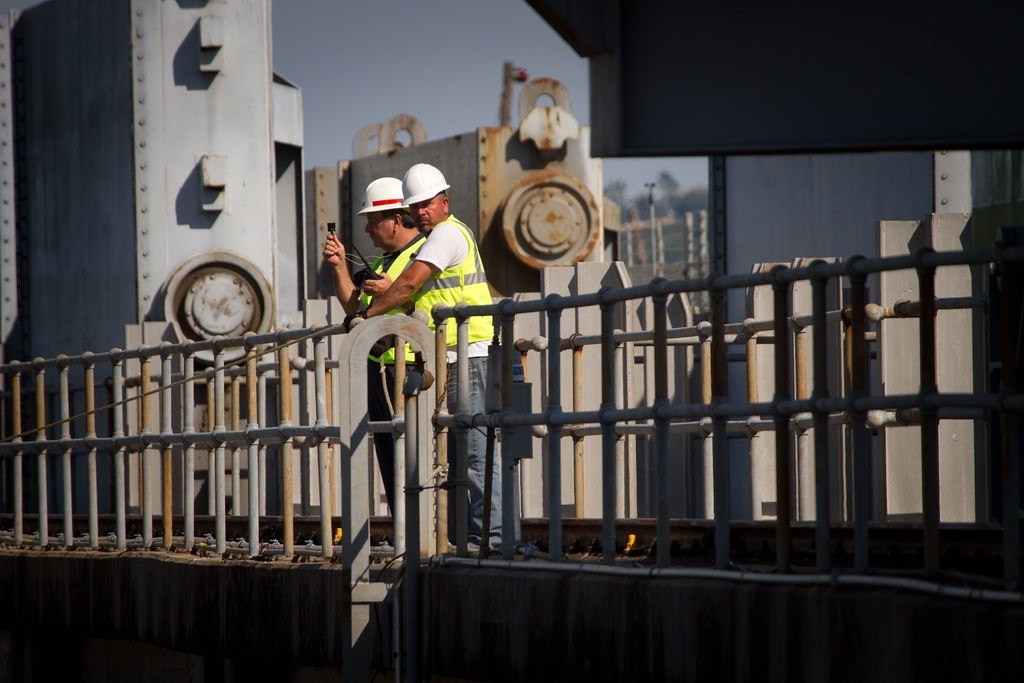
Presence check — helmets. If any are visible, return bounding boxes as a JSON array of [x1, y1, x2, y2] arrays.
[[355, 177, 410, 216], [400, 163, 450, 206]]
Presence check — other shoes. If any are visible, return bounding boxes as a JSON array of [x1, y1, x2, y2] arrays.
[[451, 541, 504, 558]]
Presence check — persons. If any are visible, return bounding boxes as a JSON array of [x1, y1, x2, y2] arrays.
[[343, 163, 521, 554], [322, 177, 428, 551]]
